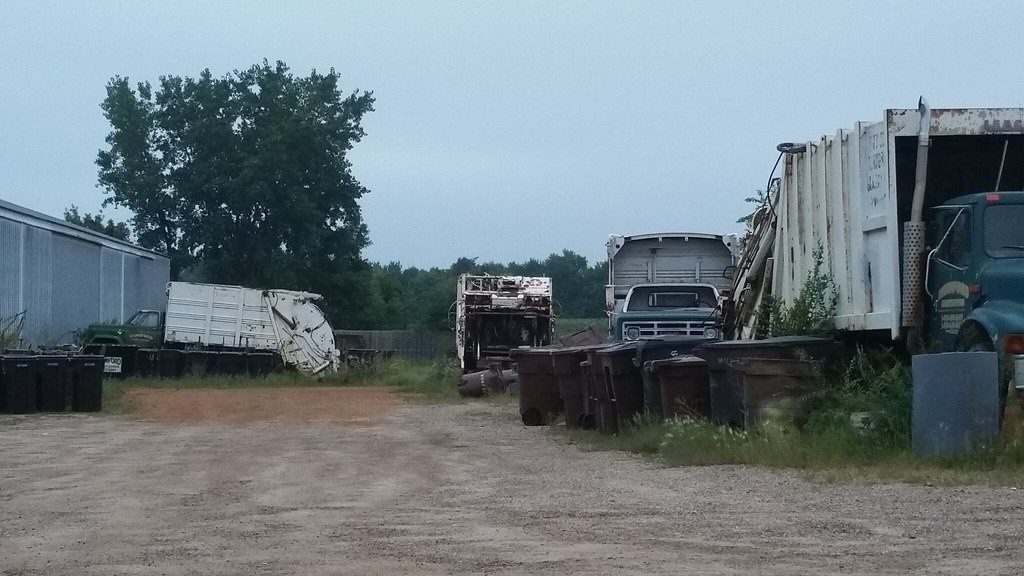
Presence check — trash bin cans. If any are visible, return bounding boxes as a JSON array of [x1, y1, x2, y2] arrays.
[[550, 335, 834, 438], [72, 354, 105, 412], [0, 354, 38, 414], [36, 355, 70, 412], [84, 344, 275, 380], [509, 347, 562, 426]]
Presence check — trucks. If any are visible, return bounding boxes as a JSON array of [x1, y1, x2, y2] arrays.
[[605, 233, 739, 343], [739, 94, 1024, 435], [77, 281, 341, 378]]
